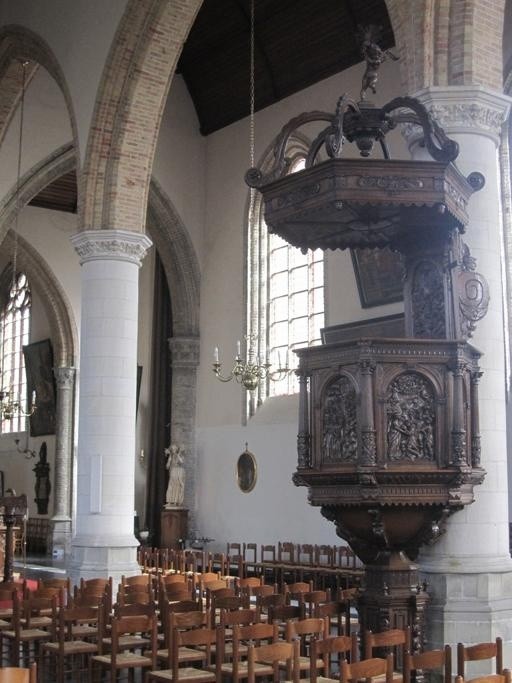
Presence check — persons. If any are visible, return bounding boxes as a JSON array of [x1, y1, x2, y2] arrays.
[[166, 444, 186, 506], [389, 386, 437, 462], [323, 383, 357, 461]]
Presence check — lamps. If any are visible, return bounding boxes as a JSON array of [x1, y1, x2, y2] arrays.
[[211, 1, 291, 390], [0, 58, 39, 418]]
